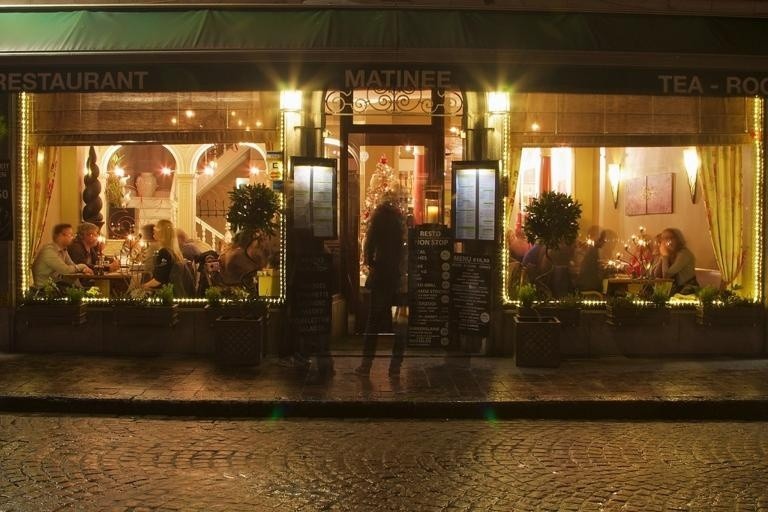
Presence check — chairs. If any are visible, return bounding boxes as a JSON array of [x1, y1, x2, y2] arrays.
[[695, 266, 723, 290]]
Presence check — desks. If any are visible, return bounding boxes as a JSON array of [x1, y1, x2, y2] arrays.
[[603, 275, 672, 294], [64, 269, 144, 298]]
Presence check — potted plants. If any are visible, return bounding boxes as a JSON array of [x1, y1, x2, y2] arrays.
[[23, 276, 269, 367], [512, 279, 761, 370]]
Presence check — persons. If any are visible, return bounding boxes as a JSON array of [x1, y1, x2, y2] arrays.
[[350, 202, 407, 377], [30, 218, 282, 298], [508, 226, 701, 296]]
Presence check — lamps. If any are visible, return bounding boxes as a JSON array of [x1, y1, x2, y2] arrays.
[[487, 91, 511, 114], [607, 225, 655, 279], [279, 88, 305, 113], [95, 233, 149, 275]]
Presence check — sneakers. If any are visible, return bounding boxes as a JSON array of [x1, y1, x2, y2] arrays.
[[389, 368, 400, 378], [353, 367, 370, 376]]
